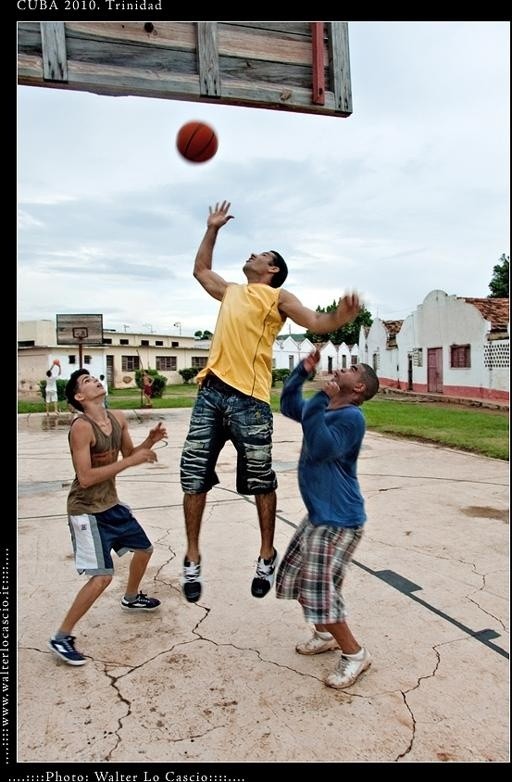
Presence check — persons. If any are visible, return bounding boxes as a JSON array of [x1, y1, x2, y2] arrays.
[[274, 346, 379, 691], [46, 362, 61, 418], [98, 374, 108, 409], [44, 368, 169, 668], [139, 368, 155, 405], [180, 198, 361, 601]]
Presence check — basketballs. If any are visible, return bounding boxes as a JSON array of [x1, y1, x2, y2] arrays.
[[174, 122, 219, 164]]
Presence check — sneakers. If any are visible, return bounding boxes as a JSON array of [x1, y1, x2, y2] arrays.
[[183, 555, 202, 602], [48, 636, 86, 666], [296, 627, 341, 655], [121, 590, 161, 611], [251, 547, 278, 598], [325, 647, 372, 689]]
